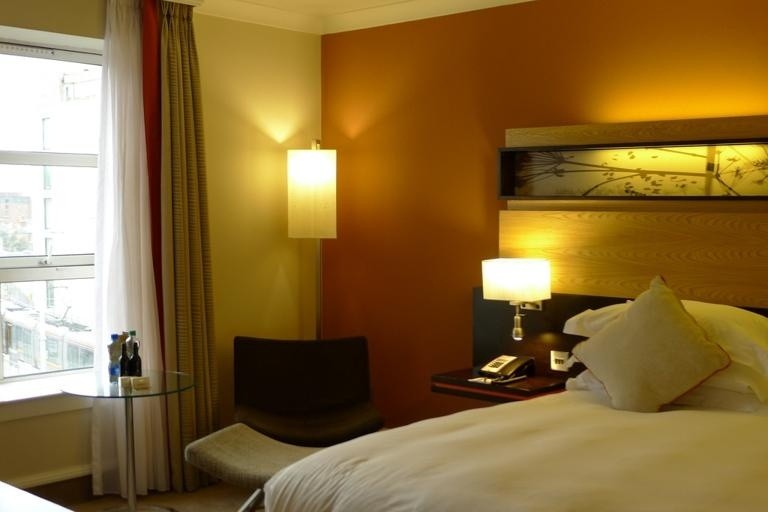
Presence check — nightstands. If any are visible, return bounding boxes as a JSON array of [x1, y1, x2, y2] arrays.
[[432, 365, 563, 401]]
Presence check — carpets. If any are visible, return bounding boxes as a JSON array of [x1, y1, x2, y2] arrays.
[[73, 482, 263, 512]]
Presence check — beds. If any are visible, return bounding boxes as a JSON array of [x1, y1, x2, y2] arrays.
[[268, 113, 768, 510]]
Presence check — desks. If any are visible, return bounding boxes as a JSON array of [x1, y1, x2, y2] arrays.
[[61, 372, 197, 512]]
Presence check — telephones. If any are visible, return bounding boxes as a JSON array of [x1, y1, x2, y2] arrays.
[[477, 352, 536, 385]]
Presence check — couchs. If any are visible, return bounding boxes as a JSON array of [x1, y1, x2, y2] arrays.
[[234, 336, 384, 445], [185, 422, 326, 512]]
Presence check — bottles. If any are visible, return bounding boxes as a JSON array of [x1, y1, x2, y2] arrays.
[[118, 330, 128, 346], [129, 342, 142, 376], [108, 334, 121, 384], [119, 342, 128, 377], [125, 330, 139, 358]]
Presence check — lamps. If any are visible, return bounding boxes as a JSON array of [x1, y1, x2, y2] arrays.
[[481, 257, 551, 343], [286, 140, 338, 341]]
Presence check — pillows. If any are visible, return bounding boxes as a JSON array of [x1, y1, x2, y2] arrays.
[[571, 274, 732, 410], [563, 298, 768, 401], [566, 370, 758, 410]]
